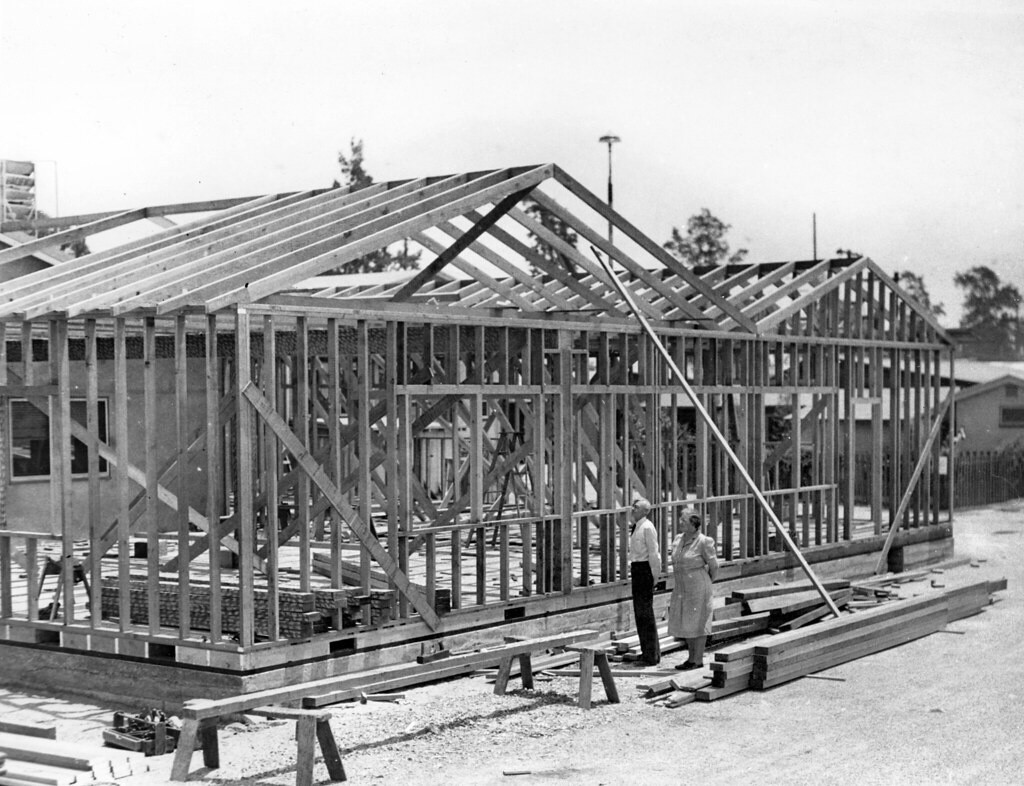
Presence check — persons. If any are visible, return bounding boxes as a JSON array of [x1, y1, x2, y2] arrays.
[[628, 498, 662, 667], [667, 509, 719, 670]]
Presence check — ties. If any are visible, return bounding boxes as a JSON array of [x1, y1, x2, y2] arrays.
[[631, 524, 636, 535]]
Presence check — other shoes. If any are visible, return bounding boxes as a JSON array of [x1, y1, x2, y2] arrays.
[[634, 659, 657, 666], [637, 654, 660, 663], [696, 662, 704, 668], [675, 660, 696, 669]]
